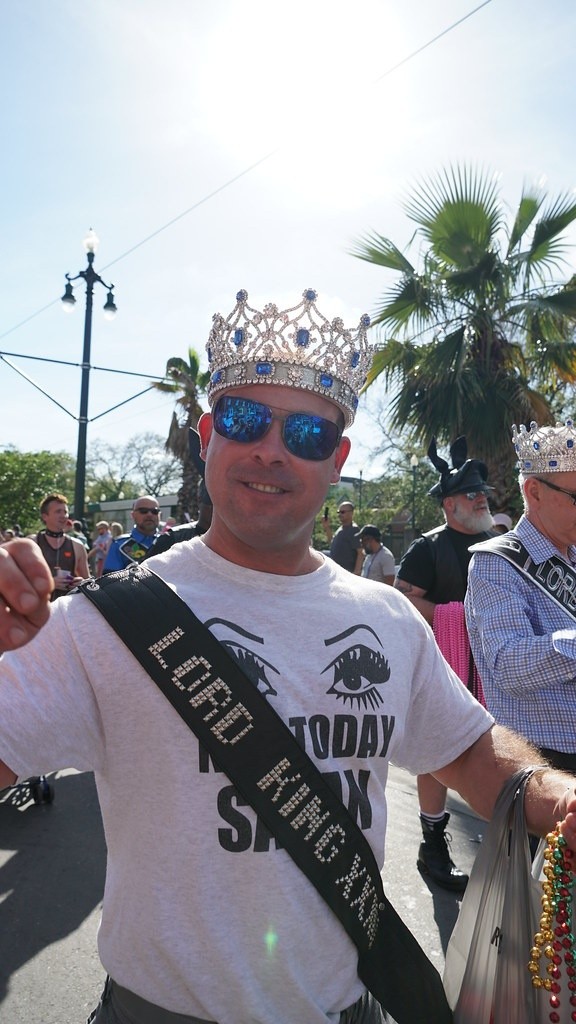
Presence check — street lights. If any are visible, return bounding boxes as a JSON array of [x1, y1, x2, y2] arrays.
[[60, 227, 119, 533], [357, 462, 365, 512], [410, 453, 420, 532]]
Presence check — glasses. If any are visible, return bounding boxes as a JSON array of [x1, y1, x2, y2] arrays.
[[462, 490, 490, 500], [212, 395, 342, 461], [336, 510, 353, 514], [534, 476, 576, 504], [134, 507, 161, 514]]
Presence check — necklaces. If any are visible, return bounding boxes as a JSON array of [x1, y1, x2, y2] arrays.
[[527, 822, 576, 1024], [433, 602, 486, 710], [46, 527, 63, 537]]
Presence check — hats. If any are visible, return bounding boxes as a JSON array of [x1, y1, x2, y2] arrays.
[[428, 432, 495, 506], [354, 524, 382, 538]]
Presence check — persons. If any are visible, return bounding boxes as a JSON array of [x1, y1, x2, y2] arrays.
[[0, 477, 213, 602], [354, 525, 396, 586], [0, 288, 576, 1024], [490, 513, 512, 534], [393, 434, 488, 891], [321, 501, 364, 576], [464, 418, 576, 868]]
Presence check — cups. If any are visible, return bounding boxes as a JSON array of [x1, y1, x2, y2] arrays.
[[57, 570, 70, 590]]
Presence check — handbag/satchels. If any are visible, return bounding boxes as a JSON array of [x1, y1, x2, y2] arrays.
[[443, 765, 576, 1024]]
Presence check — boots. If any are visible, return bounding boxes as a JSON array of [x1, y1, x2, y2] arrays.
[[416, 813, 468, 890]]
